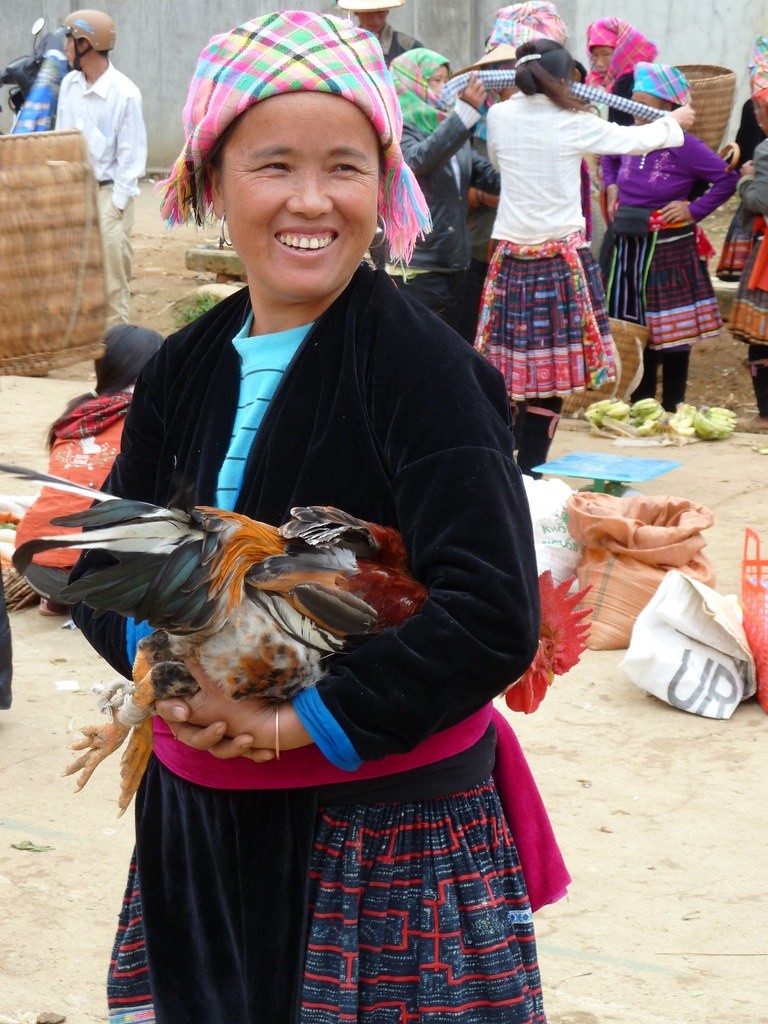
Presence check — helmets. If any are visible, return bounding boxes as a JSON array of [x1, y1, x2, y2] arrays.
[[63, 10, 116, 51]]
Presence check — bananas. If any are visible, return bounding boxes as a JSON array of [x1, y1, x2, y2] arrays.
[[584, 398, 738, 440]]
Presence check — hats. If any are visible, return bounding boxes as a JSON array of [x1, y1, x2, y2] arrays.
[[446, 43, 517, 79], [337, 0, 404, 12]]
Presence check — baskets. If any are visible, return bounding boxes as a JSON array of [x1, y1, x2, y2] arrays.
[[676, 65, 736, 154], [561, 317, 649, 417], [742, 529, 768, 715]]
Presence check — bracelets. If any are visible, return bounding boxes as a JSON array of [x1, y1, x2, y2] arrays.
[[275, 702, 279, 759]]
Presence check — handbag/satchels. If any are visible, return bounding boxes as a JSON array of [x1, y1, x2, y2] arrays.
[[618, 570, 756, 718]]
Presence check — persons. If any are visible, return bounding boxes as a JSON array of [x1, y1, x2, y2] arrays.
[[339, 0, 422, 62], [447, 0, 594, 332], [15, 326, 165, 617], [473, 39, 695, 481], [716, 35, 768, 433], [601, 63, 740, 413], [585, 17, 658, 127], [391, 49, 500, 331], [56, 11, 149, 327], [71, 10, 573, 1024]]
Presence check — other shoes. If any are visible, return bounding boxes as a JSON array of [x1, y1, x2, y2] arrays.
[[742, 415, 768, 432]]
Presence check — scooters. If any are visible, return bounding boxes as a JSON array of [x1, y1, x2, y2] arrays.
[[0, 18, 71, 134]]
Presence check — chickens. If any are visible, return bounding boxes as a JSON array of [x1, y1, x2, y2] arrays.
[[0, 464, 595, 820]]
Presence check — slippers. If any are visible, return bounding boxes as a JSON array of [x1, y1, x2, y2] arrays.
[[40, 598, 60, 616]]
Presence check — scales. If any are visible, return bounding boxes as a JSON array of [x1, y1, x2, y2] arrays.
[[531, 451, 682, 499]]
